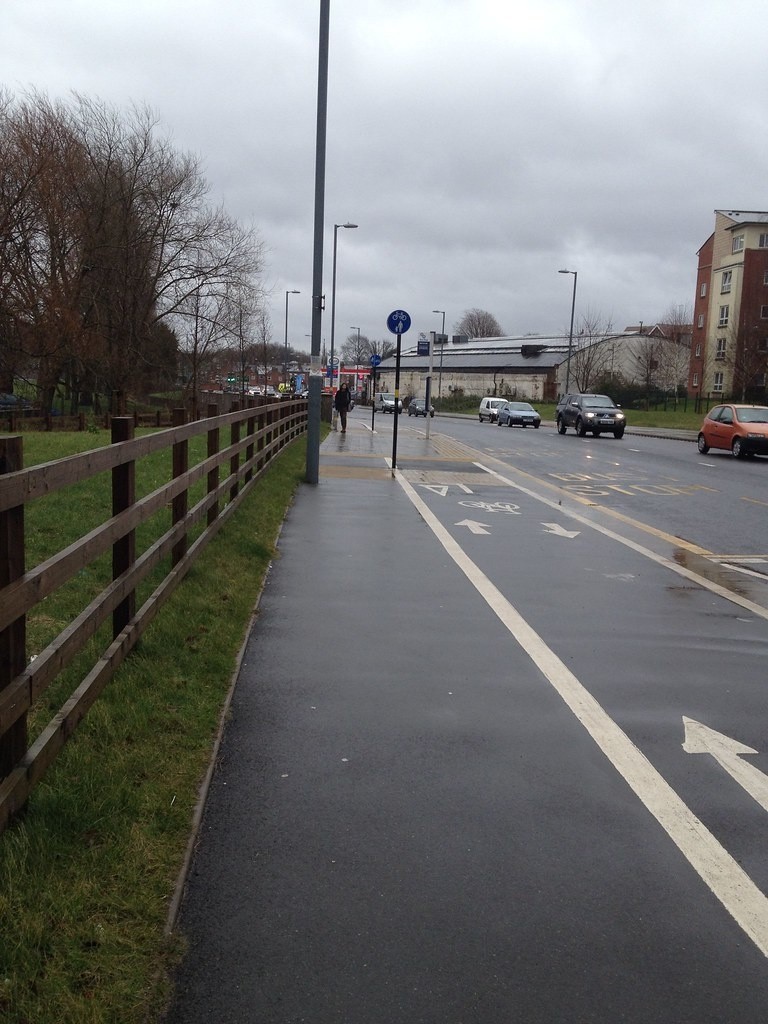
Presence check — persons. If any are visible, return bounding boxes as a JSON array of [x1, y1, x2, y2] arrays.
[[336, 383, 352, 433], [362, 390, 367, 405]]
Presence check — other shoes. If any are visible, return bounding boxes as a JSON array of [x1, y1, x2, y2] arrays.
[[341, 430, 346, 433]]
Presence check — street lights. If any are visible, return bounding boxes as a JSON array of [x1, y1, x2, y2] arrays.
[[430, 331, 437, 344], [330, 222, 359, 385], [639, 321, 644, 336], [432, 310, 446, 410], [558, 270, 577, 395], [348, 325, 361, 400], [283, 290, 301, 391]]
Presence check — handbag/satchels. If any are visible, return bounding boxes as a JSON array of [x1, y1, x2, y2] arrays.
[[348, 400, 354, 411]]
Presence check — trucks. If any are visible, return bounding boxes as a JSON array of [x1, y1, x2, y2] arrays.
[[375, 392, 403, 414]]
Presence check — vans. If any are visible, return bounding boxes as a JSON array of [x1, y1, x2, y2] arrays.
[[478, 397, 508, 423]]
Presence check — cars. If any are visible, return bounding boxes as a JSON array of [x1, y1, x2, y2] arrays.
[[408, 399, 435, 417], [495, 401, 542, 428], [249, 388, 310, 397], [557, 394, 627, 438], [697, 403, 768, 457], [554, 393, 574, 420]]
